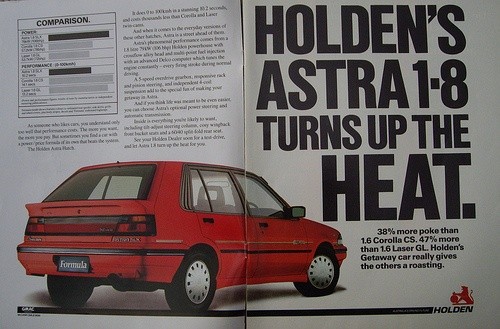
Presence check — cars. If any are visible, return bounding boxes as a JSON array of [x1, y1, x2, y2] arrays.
[[17, 161, 348, 315]]
